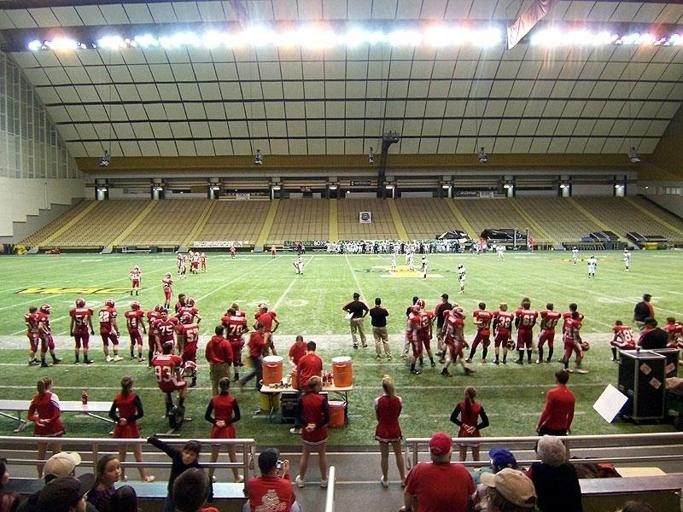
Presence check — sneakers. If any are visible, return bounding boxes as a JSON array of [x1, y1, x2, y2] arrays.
[[137, 357, 146, 362], [113, 354, 124, 362], [318, 474, 329, 488], [295, 473, 306, 489], [29, 360, 40, 366], [234, 474, 246, 483], [159, 371, 264, 424], [379, 475, 390, 488], [352, 342, 592, 377], [40, 362, 52, 367], [105, 354, 115, 363], [141, 475, 156, 483], [399, 474, 408, 487], [83, 359, 95, 363], [52, 357, 63, 365], [129, 354, 137, 360], [176, 267, 210, 281]]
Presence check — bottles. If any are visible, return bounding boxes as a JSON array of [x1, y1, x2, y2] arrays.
[[321, 371, 333, 387], [273, 380, 288, 389], [81, 391, 87, 405]]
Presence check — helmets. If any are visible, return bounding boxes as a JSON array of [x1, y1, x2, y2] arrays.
[[104, 298, 115, 308], [505, 340, 517, 352], [410, 298, 465, 319], [580, 341, 592, 352], [129, 301, 141, 311], [153, 297, 198, 371], [73, 297, 86, 308], [39, 303, 53, 315]]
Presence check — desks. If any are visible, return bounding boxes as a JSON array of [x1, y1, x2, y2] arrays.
[[0, 478, 249, 512], [0, 398, 120, 435], [260, 377, 354, 424], [577, 473, 682, 510]]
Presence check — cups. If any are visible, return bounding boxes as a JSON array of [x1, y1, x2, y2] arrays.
[[635, 346, 642, 353]]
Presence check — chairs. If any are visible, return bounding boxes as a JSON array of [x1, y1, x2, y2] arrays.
[[14, 197, 682, 248]]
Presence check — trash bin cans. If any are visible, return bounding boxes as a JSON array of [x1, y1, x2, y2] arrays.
[[258, 380, 283, 410]]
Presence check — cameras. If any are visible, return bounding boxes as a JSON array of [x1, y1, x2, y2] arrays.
[[276, 460, 284, 468]]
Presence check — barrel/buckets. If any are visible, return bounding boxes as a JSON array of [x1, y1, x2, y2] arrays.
[[328, 400, 347, 426], [332, 356, 353, 387], [259, 379, 279, 413], [262, 355, 283, 384]]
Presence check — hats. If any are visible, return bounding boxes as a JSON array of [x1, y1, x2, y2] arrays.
[[429, 432, 453, 457], [41, 451, 81, 486], [489, 448, 517, 471], [478, 466, 539, 508], [535, 433, 568, 470], [258, 448, 279, 471], [36, 473, 96, 512]]
